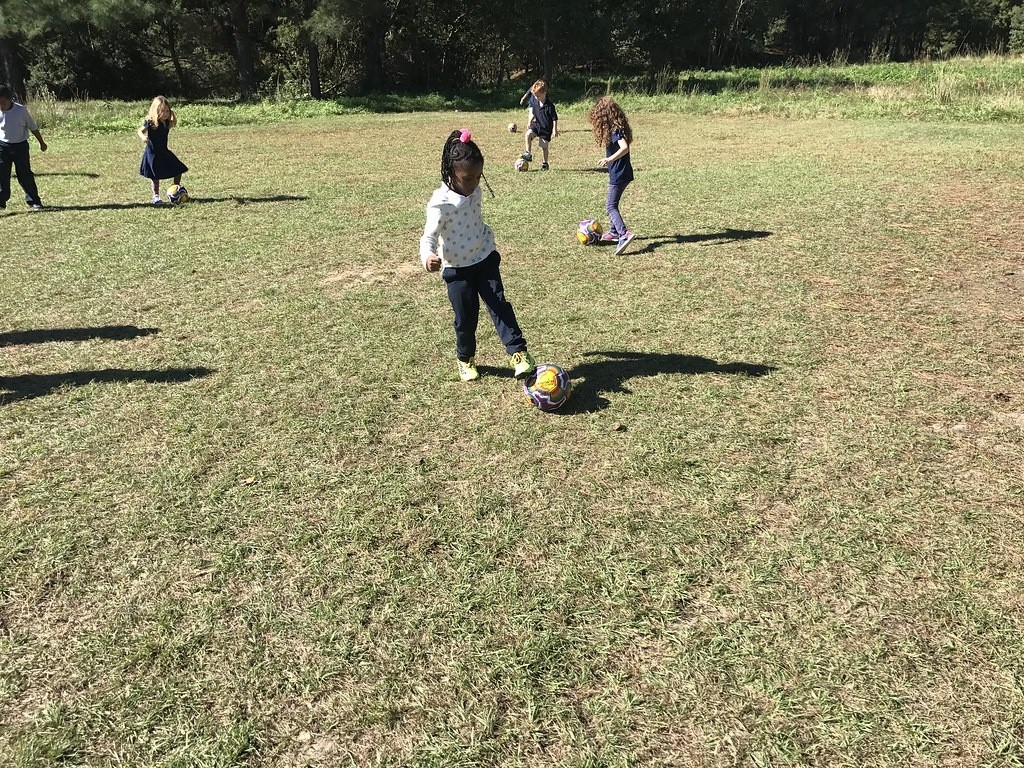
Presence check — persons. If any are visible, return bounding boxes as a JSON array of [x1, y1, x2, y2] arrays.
[[138, 96, 190, 206], [419, 130, 535, 382], [0, 84, 48, 212], [590, 96, 635, 255], [518, 79, 560, 171]]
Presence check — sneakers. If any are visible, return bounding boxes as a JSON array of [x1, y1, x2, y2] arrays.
[[510, 351, 535, 379], [541, 163, 549, 171], [600, 232, 619, 242], [457, 356, 479, 382], [613, 230, 635, 254], [519, 151, 533, 162]]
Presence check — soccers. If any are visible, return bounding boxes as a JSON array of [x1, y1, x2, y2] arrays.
[[577, 219, 603, 245], [524, 364, 571, 412], [515, 158, 529, 173], [508, 123, 517, 132], [168, 184, 188, 205]]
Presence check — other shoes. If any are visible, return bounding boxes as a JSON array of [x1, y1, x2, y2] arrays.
[[0, 206, 6, 210], [153, 194, 164, 205], [30, 202, 44, 211]]
